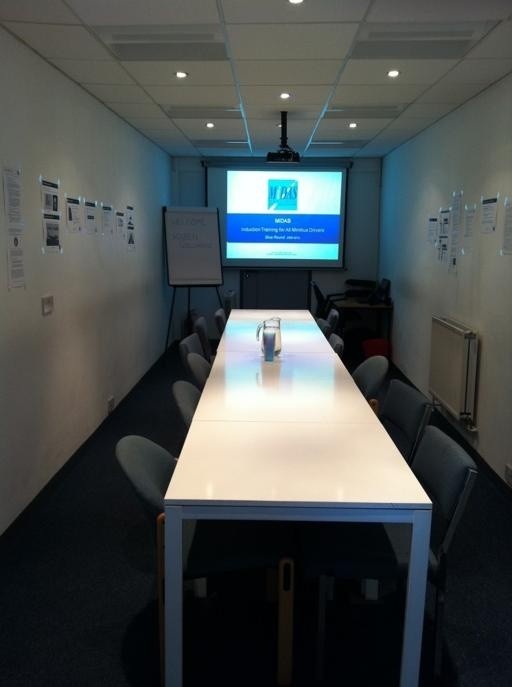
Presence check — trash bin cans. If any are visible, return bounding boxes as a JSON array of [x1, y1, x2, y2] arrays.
[[364, 339, 390, 357]]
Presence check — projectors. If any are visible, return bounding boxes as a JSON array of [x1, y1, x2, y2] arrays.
[[264, 149, 301, 164]]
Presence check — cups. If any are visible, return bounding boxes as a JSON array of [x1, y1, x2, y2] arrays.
[[262, 331, 276, 361]]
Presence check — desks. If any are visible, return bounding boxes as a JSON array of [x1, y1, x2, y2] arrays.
[[163, 309, 434, 686], [329, 293, 393, 338]]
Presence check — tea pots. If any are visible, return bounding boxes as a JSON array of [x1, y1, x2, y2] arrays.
[[256, 316, 282, 355]]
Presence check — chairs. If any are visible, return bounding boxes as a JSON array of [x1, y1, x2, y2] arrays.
[[311, 280, 327, 319], [114, 311, 293, 684], [307, 354, 479, 684], [318, 310, 342, 353]]
